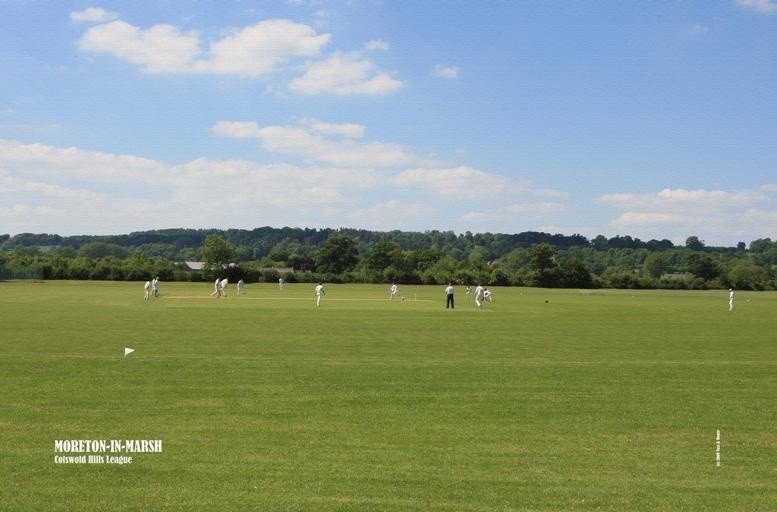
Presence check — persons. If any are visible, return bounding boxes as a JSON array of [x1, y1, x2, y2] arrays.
[[483, 291, 493, 298], [727, 287, 734, 311], [483, 288, 490, 302], [143, 278, 151, 304], [210, 276, 221, 298], [237, 278, 245, 296], [464, 284, 471, 296], [151, 276, 156, 297], [444, 282, 454, 309], [474, 282, 483, 306], [278, 277, 284, 289], [218, 276, 230, 297], [154, 276, 159, 296], [390, 282, 400, 301], [314, 282, 324, 308]]
